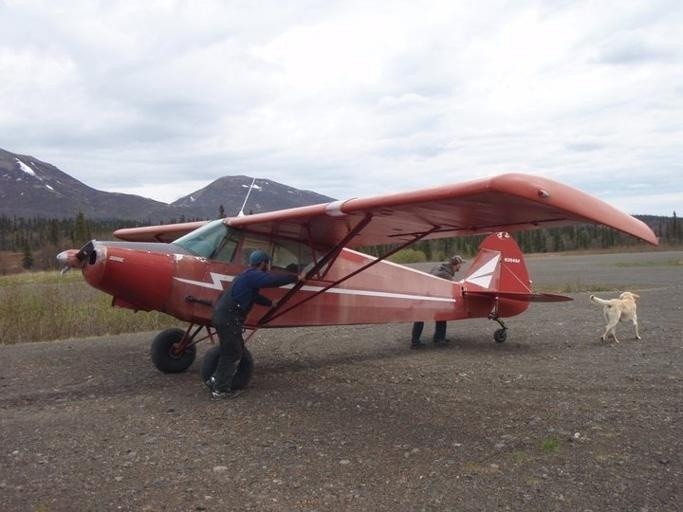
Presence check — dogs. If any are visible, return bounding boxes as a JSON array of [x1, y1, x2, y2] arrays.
[[590, 292, 642, 345]]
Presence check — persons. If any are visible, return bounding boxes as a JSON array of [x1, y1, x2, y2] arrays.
[[409, 256, 468, 347], [204, 250, 307, 400]]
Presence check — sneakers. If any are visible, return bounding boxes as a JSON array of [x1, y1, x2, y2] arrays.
[[434, 337, 448, 343], [411, 341, 424, 346], [205, 376, 240, 400]]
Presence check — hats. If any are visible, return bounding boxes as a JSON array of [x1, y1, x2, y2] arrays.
[[450, 256, 466, 265], [250, 251, 272, 265]]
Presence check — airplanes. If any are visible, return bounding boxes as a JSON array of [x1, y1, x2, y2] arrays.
[[57, 173, 660, 390]]
